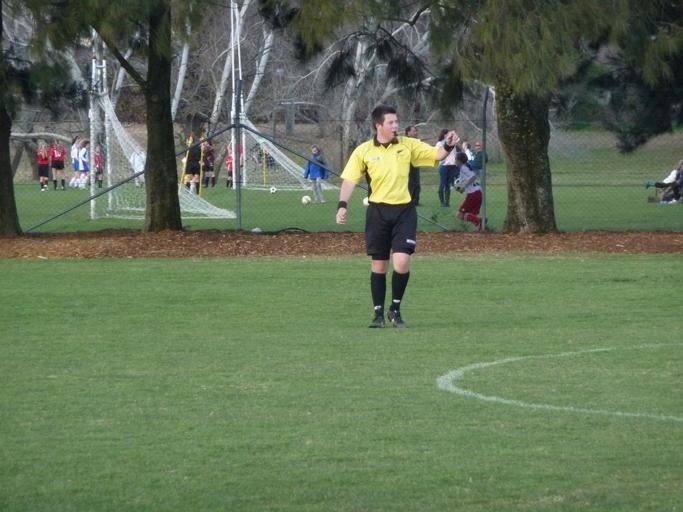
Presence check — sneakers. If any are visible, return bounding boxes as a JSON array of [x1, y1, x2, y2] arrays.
[[203, 184, 215, 188], [41, 184, 65, 191], [387, 303, 404, 327], [480, 217, 487, 231], [369, 308, 385, 328], [69, 183, 88, 190]]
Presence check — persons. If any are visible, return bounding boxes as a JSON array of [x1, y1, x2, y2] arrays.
[[405, 126, 489, 232], [671, 159, 683, 202], [304, 144, 329, 203], [336, 105, 460, 328], [37, 136, 105, 191], [130, 146, 146, 188], [184, 128, 244, 197]]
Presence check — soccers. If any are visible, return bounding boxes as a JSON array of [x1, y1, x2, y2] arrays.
[[269, 187, 277, 194], [453, 178, 466, 191], [363, 197, 370, 206], [302, 195, 311, 205]]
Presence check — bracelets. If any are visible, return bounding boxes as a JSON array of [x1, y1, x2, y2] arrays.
[[338, 201, 347, 209]]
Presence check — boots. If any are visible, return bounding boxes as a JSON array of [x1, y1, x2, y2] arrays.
[[439, 190, 450, 207]]
